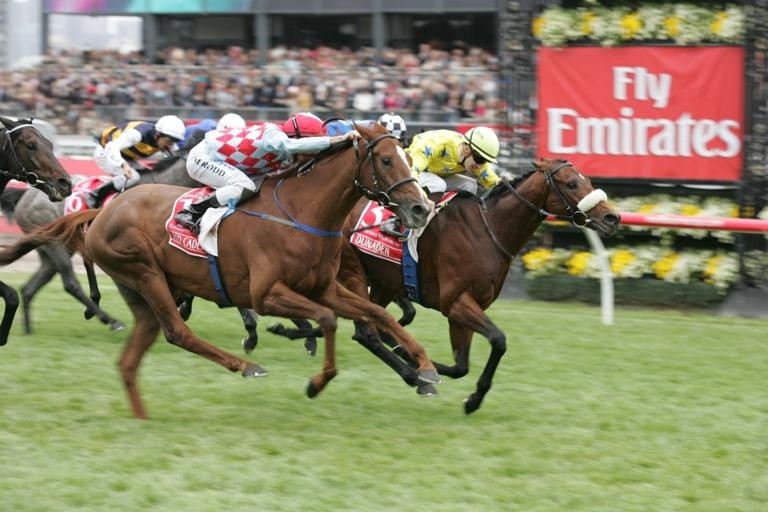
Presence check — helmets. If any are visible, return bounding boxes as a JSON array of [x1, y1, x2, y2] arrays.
[[374, 110, 409, 141], [459, 125, 501, 164], [153, 114, 189, 142], [215, 112, 245, 134], [282, 110, 325, 139]]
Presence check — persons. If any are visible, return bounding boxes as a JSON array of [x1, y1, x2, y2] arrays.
[[326, 113, 407, 142], [402, 126, 501, 303], [173, 113, 246, 152], [82, 115, 186, 209], [174, 112, 362, 235], [1, 43, 498, 117]]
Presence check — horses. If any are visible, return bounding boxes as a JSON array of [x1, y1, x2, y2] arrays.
[[0, 116, 622, 420]]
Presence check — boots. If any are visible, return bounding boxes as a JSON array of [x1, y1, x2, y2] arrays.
[[84, 179, 123, 212], [174, 190, 222, 236]]
